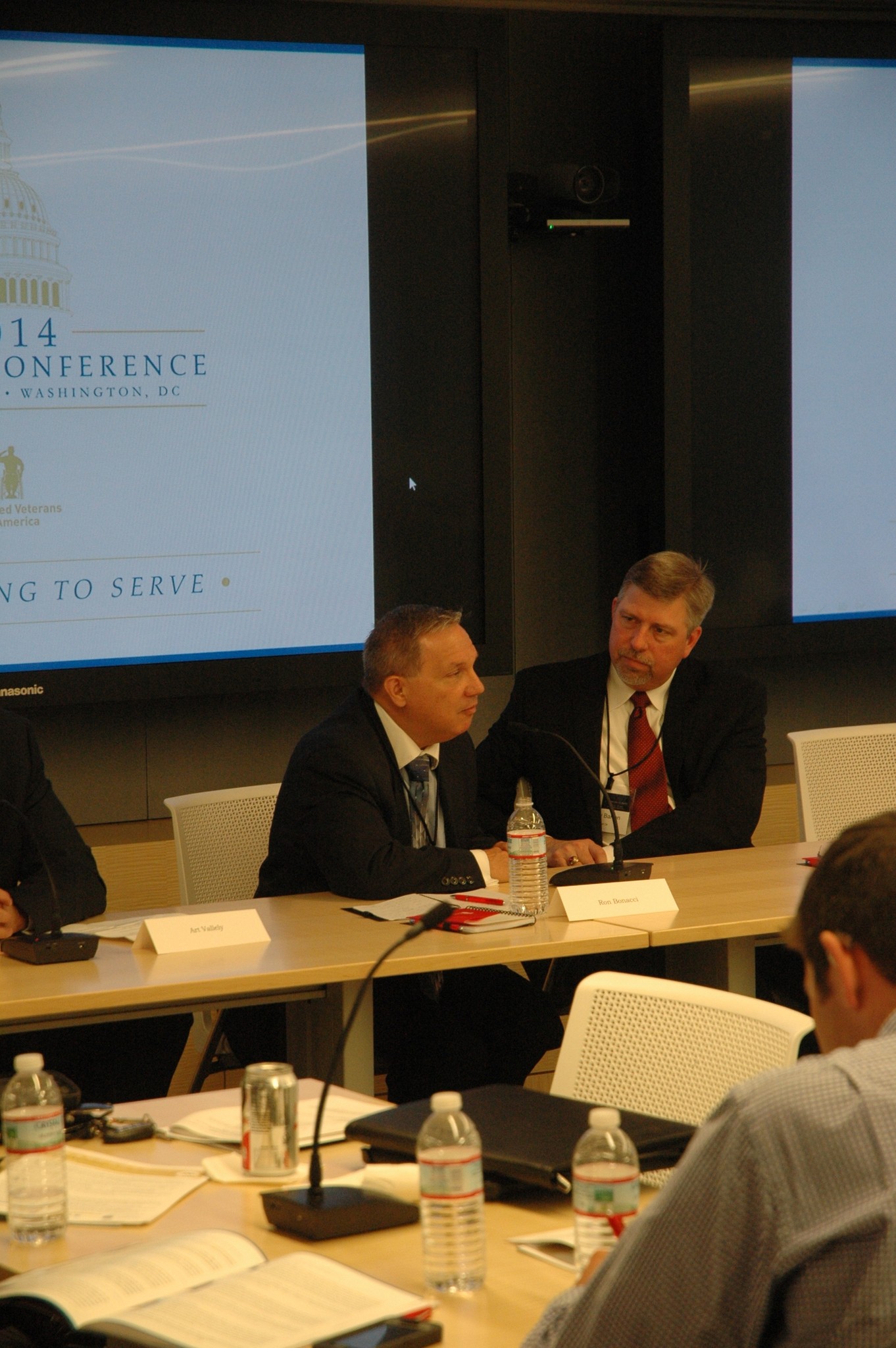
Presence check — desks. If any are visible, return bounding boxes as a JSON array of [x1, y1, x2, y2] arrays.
[[0, 1078, 674, 1348], [0, 843, 825, 1098]]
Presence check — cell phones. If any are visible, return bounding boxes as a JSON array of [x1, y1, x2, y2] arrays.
[[315, 1319, 442, 1348]]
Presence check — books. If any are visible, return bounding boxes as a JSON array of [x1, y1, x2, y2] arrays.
[[0, 1227, 441, 1348]]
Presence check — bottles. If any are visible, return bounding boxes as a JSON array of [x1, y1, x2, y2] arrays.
[[415, 1091, 486, 1297], [572, 1105, 640, 1277], [2, 1053, 67, 1247], [507, 797, 548, 915]]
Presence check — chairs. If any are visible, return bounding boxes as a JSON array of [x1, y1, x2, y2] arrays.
[[163, 783, 281, 1099], [549, 971, 815, 1129], [787, 723, 896, 842]]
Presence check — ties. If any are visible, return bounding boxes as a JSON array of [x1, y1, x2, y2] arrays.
[[627, 688, 673, 834], [406, 752, 431, 850]]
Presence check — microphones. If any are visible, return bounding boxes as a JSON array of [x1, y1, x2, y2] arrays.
[[259, 902, 454, 1238], [504, 723, 653, 886]]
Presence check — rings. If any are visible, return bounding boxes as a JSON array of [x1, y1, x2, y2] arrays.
[[568, 856, 579, 865]]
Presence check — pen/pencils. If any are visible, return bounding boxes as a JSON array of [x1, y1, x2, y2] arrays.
[[450, 894, 505, 906]]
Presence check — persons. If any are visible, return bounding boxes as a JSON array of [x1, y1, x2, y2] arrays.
[[523, 808, 896, 1348], [221, 604, 552, 1104], [0, 706, 193, 1108], [475, 550, 822, 1057]]
